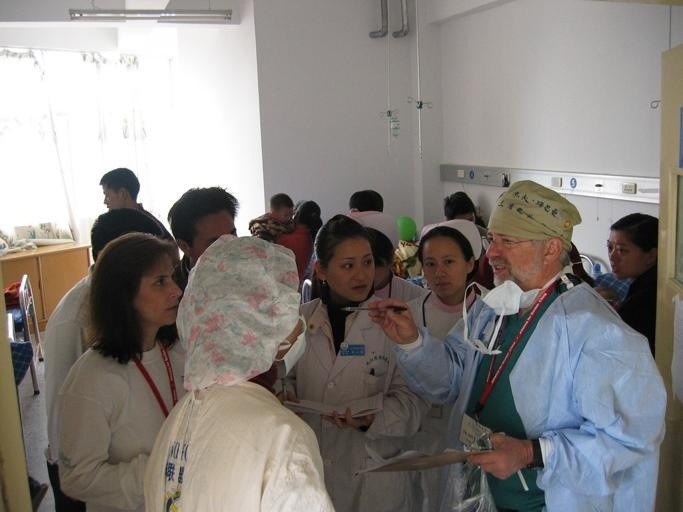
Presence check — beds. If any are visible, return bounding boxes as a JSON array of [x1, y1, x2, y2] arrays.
[[5, 272, 44, 397]]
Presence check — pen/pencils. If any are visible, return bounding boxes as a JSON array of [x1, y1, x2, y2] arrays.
[[280, 377, 288, 401], [340, 305, 408, 313]]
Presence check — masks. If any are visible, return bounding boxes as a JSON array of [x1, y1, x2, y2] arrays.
[[273, 314, 308, 380], [462, 264, 573, 355]]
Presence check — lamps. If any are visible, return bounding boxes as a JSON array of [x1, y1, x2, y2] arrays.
[[67, 1, 235, 24]]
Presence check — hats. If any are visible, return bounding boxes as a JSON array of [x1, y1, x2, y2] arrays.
[[420, 219, 483, 261], [346, 209, 401, 248], [486, 179, 582, 251]]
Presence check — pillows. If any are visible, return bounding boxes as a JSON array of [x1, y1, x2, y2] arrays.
[[8, 340, 33, 385]]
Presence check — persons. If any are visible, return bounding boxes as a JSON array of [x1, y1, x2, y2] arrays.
[[44, 168, 659, 512]]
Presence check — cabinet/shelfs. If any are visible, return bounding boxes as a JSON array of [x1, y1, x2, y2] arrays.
[[0, 241, 92, 331]]
